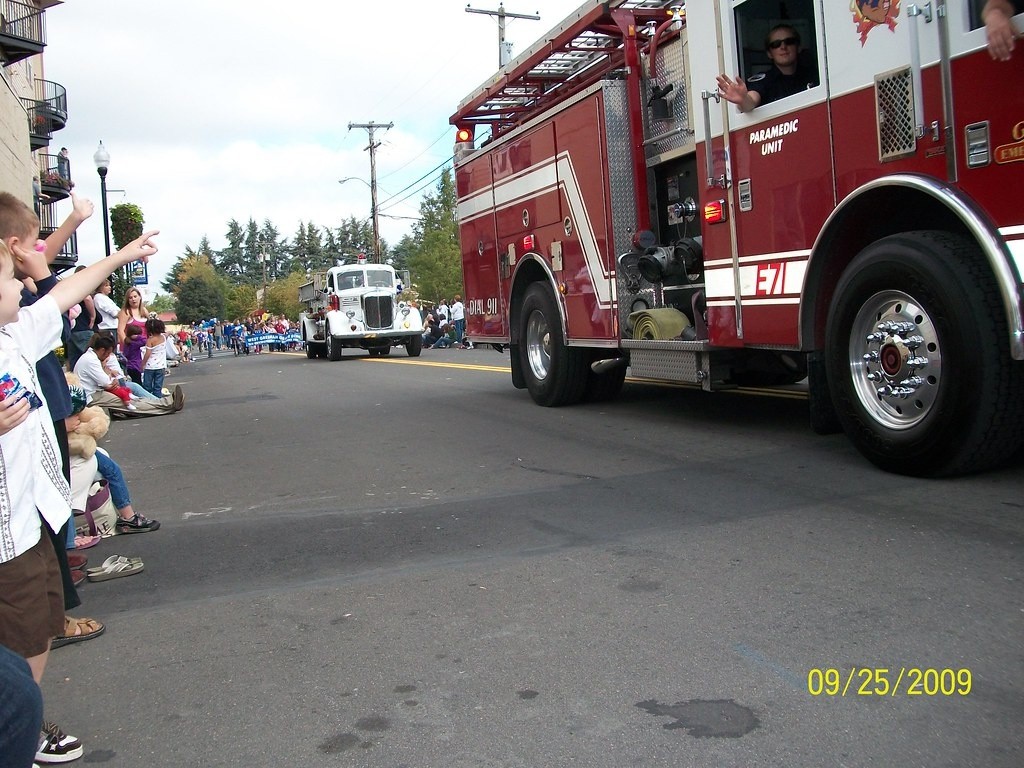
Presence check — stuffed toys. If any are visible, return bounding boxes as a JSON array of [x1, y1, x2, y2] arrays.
[[64, 372, 111, 458]]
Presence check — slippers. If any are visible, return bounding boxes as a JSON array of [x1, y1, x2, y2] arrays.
[[87, 554, 144, 582]]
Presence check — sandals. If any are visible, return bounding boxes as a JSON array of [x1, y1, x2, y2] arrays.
[[50, 616, 106, 650], [74, 536, 101, 550]]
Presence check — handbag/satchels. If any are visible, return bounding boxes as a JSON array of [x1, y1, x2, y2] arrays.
[[70, 479, 121, 538]]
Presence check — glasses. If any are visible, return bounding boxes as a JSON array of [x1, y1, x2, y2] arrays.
[[769, 37, 795, 49]]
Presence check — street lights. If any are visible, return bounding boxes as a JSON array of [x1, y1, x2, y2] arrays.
[[338, 176, 381, 264], [93, 139, 115, 300]]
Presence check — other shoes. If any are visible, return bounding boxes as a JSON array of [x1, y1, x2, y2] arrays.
[[68, 570, 86, 586], [172, 385, 185, 411], [66, 551, 87, 571], [457, 345, 474, 350]]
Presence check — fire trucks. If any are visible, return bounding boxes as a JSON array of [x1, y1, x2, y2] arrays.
[[448, 0, 1024, 481]]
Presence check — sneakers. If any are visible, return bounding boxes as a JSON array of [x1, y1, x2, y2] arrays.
[[32, 720, 83, 763], [116, 510, 160, 534]]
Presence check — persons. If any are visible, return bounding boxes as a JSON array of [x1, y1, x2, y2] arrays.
[[980, 0, 1024, 61], [0, 147, 474, 768], [716, 22, 820, 114]]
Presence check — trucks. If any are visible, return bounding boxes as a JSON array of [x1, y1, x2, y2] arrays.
[[299, 251, 427, 361]]
